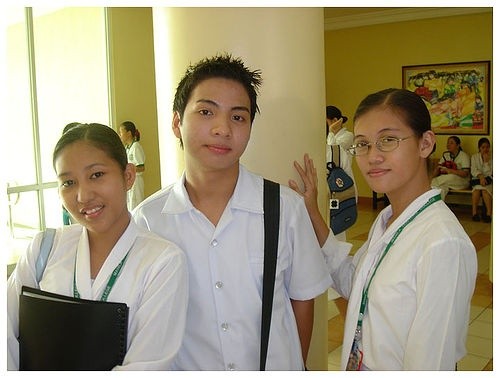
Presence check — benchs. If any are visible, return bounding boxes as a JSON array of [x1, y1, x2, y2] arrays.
[[429, 157, 483, 206]]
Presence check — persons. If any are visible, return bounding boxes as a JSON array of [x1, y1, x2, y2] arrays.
[[430, 136, 471, 201], [288, 88, 478, 371], [7, 122, 189, 371], [118, 121, 146, 225], [470, 138, 493, 223], [326, 119, 358, 242], [130, 55, 334, 371], [326, 106, 354, 165]]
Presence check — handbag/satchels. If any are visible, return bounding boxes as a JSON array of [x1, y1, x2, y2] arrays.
[[468, 176, 492, 187], [440, 151, 457, 175], [326, 161, 358, 235]]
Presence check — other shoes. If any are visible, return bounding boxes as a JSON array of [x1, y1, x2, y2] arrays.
[[484, 215, 491, 223], [472, 214, 480, 221]]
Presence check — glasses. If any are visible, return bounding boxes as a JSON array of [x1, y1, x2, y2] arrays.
[[348, 135, 412, 156]]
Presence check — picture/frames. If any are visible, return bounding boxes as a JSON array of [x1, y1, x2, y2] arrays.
[[401, 60, 490, 136]]
